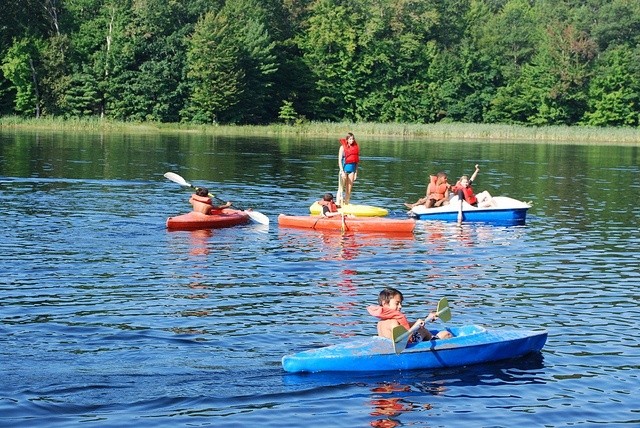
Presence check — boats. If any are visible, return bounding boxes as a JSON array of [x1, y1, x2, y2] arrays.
[[407, 197, 532, 226], [309, 201, 388, 218], [278, 213, 416, 232], [282, 323, 548, 373], [166, 208, 252, 231]]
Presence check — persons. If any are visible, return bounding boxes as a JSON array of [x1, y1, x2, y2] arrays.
[[404, 172, 449, 208], [322, 193, 342, 218], [336, 132, 359, 205], [189, 187, 253, 216], [376, 287, 454, 338], [457, 163, 496, 223]]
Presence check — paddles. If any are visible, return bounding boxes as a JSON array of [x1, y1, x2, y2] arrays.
[[392, 298, 452, 354], [339, 172, 345, 237], [163, 172, 269, 226]]
